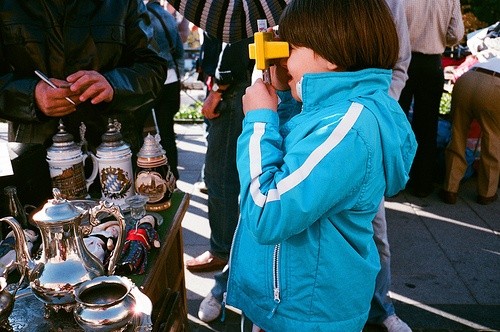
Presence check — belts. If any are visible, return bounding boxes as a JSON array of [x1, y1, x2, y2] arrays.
[[472, 67, 500, 77]]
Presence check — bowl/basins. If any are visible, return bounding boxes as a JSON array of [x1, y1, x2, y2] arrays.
[[72, 275, 137, 332]]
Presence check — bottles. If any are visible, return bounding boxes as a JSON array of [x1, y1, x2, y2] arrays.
[[2, 185, 27, 235], [95, 117, 135, 213], [134, 132, 177, 212], [45, 118, 99, 200]]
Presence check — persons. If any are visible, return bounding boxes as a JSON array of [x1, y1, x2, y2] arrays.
[[0, 0, 187, 182], [186, 0, 500, 332], [77, 221, 119, 264], [117, 213, 162, 275]]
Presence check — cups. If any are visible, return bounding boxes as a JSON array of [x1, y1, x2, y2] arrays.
[[125, 195, 148, 219]]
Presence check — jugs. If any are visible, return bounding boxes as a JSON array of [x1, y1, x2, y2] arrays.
[[0, 188, 126, 319], [0, 260, 27, 332]]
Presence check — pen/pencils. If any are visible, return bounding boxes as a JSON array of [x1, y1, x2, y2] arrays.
[[35, 71, 75, 105]]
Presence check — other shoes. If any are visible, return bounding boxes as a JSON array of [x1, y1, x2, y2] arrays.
[[476, 193, 497, 206], [415, 184, 432, 197], [437, 189, 456, 205], [194, 181, 209, 193]]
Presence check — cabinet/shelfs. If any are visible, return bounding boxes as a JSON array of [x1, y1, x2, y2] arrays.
[[1, 191, 189, 332]]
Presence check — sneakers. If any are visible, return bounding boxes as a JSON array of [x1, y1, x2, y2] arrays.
[[198, 291, 224, 322], [186, 251, 227, 272], [383, 314, 413, 332]]
